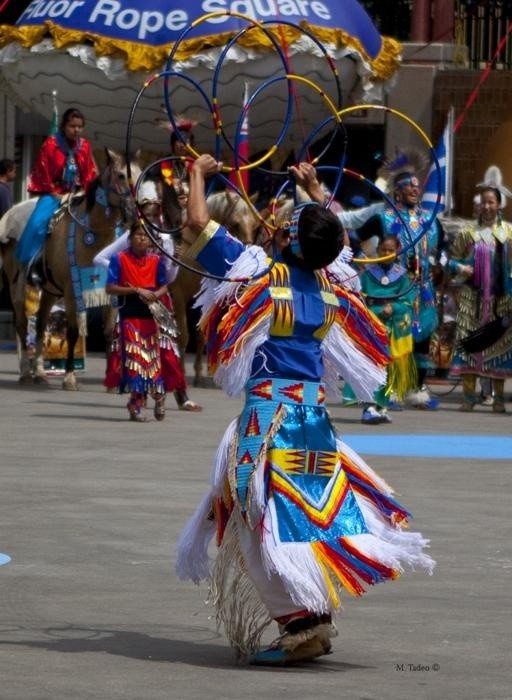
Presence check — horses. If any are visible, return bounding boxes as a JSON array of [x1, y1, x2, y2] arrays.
[[167, 189, 261, 388], [258, 182, 325, 256], [0, 147, 144, 393]]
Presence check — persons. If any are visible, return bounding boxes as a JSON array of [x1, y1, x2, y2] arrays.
[[0, 100, 512, 424], [179, 152, 422, 666]]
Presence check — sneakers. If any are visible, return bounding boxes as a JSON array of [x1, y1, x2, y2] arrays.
[[459, 401, 472, 411], [482, 397, 494, 405], [493, 403, 505, 413], [415, 400, 439, 409], [179, 400, 202, 410], [128, 394, 166, 422], [248, 632, 332, 666], [362, 403, 400, 424]]
